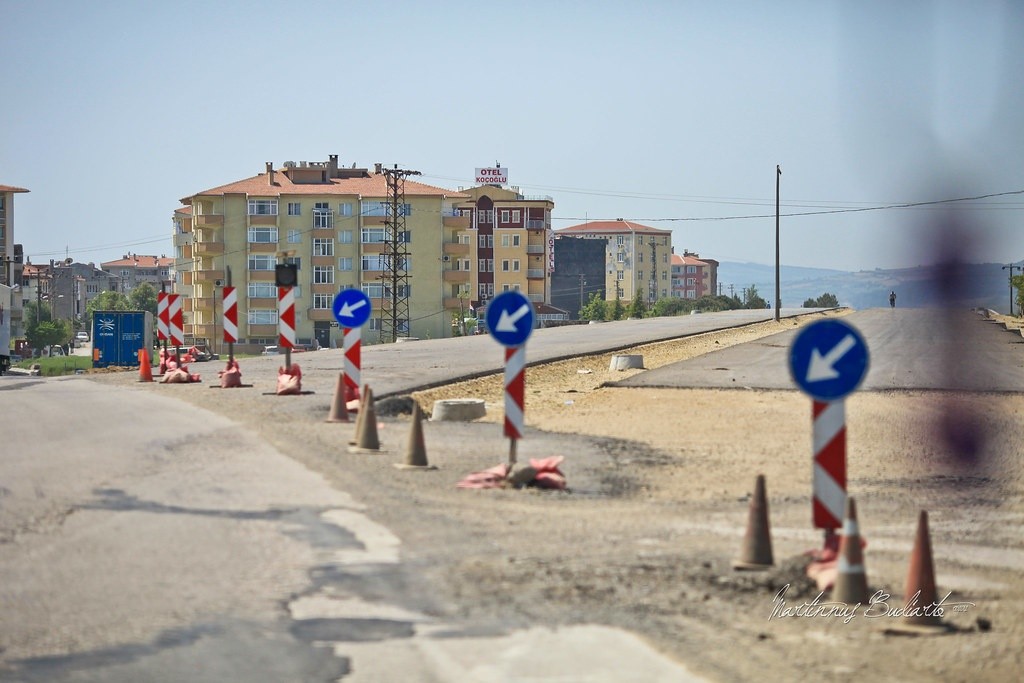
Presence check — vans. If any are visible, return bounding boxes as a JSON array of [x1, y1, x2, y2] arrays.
[[76, 332, 89, 342]]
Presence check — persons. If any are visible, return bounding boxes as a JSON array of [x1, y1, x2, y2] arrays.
[[889, 291, 896, 306]]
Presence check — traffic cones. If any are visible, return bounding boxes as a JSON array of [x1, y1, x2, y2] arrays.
[[820, 496, 884, 614], [392, 402, 438, 472], [136, 347, 157, 382], [325, 373, 350, 423], [346, 384, 387, 453], [877, 510, 955, 636], [732, 474, 777, 571]]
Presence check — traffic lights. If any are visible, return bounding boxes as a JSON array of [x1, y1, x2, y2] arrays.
[[274, 264, 297, 286]]
